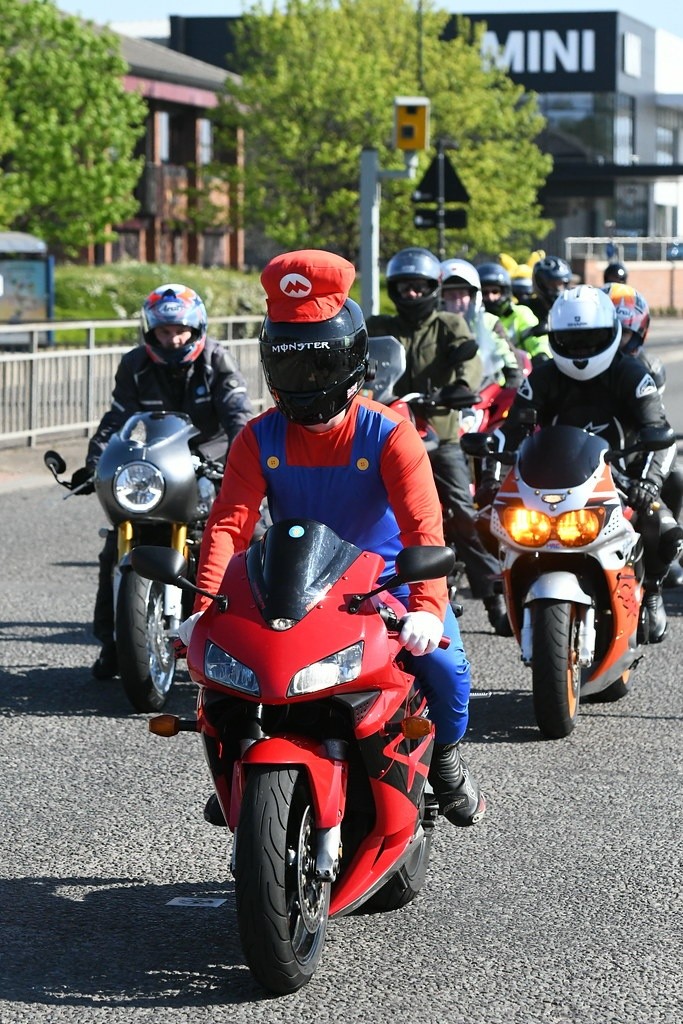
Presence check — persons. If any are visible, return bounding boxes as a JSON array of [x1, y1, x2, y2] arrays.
[[604, 265, 628, 284], [387, 247, 571, 624], [482, 282, 683, 642], [71, 284, 254, 680], [180, 256, 486, 827]]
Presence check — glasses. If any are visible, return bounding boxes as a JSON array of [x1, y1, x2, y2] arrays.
[[395, 281, 431, 293]]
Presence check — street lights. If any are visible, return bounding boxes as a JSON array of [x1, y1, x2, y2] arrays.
[[429, 138, 462, 265]]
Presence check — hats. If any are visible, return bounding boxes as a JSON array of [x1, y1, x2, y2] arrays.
[[260, 249, 355, 323]]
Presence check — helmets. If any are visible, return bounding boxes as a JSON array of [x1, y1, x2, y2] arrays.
[[475, 264, 513, 313], [139, 284, 208, 368], [259, 297, 371, 426], [602, 282, 651, 357], [541, 285, 624, 380], [513, 277, 535, 297], [603, 264, 629, 282], [438, 259, 484, 317], [532, 256, 574, 292], [384, 248, 443, 319]]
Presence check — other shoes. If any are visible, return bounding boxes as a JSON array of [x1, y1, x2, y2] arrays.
[[482, 593, 507, 628], [91, 651, 117, 681], [662, 564, 683, 589]]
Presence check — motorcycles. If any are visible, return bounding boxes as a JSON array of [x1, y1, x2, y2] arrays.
[[357, 317, 550, 615], [126, 515, 459, 996], [457, 425, 677, 740], [44, 410, 272, 714]]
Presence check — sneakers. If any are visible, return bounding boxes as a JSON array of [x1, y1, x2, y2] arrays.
[[204, 794, 228, 826], [428, 747, 487, 828], [644, 592, 668, 644]]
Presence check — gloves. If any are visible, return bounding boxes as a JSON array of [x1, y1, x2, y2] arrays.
[[475, 478, 502, 509], [178, 611, 206, 645], [70, 468, 96, 496], [400, 611, 444, 656], [439, 383, 474, 412], [627, 477, 661, 516]]
